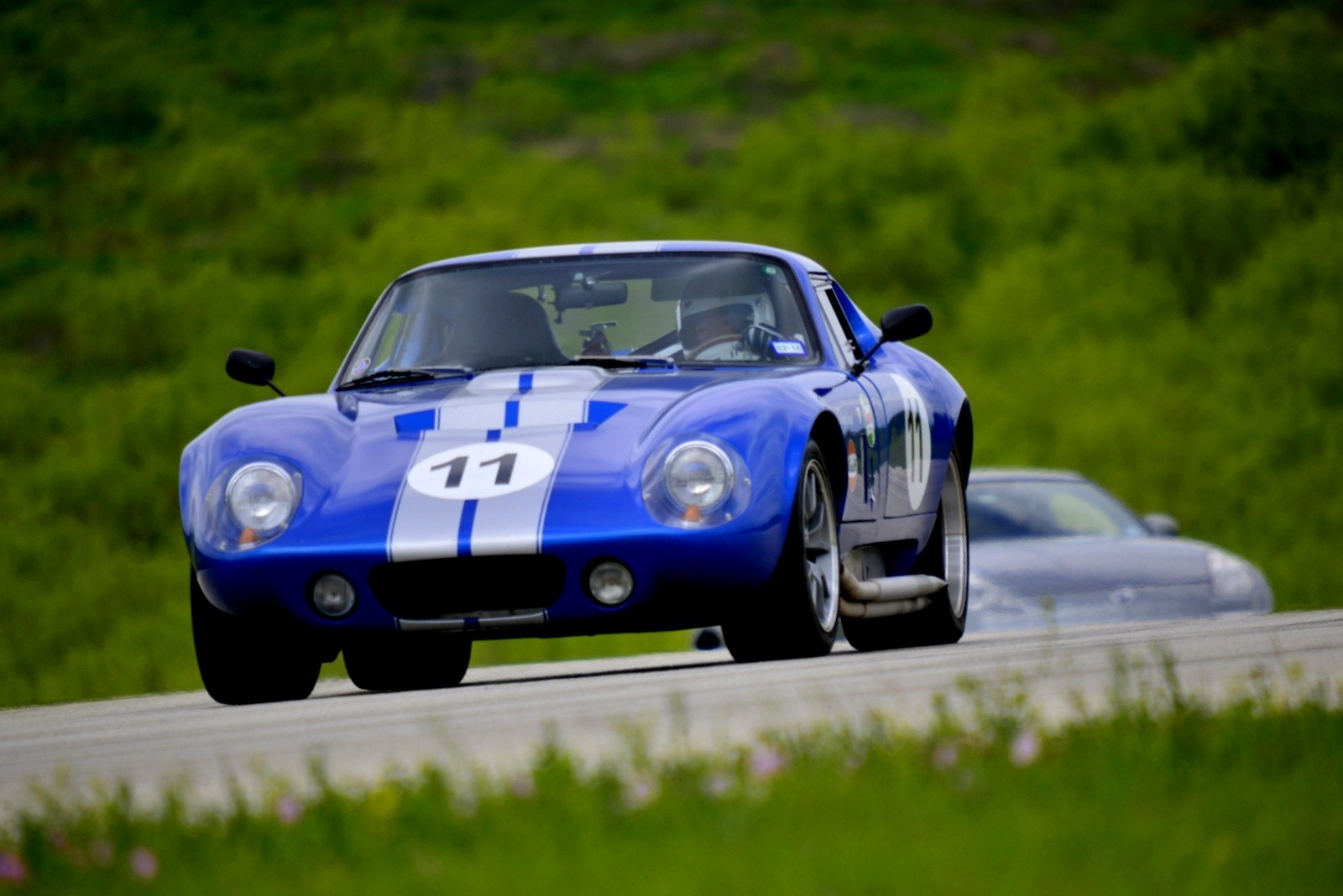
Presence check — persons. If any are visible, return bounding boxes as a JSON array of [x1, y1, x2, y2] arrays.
[[675, 290, 777, 361]]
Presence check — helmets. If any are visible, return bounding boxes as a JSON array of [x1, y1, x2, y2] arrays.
[[676, 290, 776, 362]]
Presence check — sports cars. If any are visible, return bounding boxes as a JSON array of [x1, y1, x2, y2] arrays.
[[176, 239, 976, 705], [960, 467, 1274, 636]]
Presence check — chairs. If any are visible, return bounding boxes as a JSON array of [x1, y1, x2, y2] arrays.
[[460, 293, 567, 362]]
